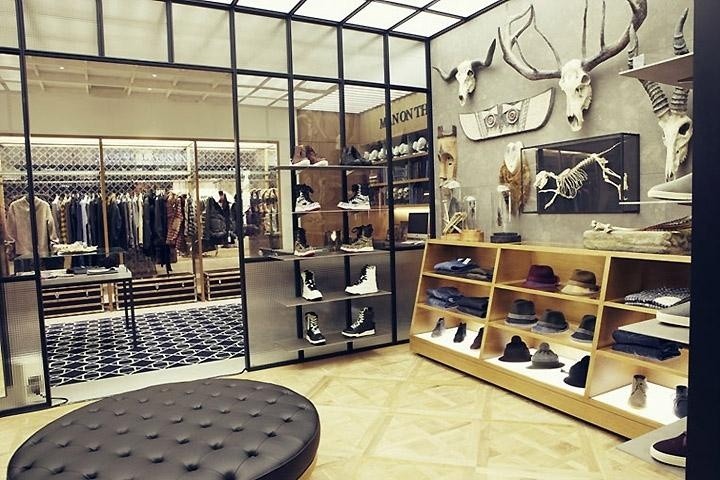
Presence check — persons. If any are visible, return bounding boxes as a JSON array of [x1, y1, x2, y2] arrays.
[[218, 190, 224, 210], [228, 193, 237, 244]]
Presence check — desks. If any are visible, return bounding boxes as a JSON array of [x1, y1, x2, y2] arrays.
[[8, 264, 137, 352]]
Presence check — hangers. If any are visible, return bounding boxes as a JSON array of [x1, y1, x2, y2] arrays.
[[10, 185, 280, 209]]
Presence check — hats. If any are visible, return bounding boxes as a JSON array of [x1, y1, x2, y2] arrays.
[[530, 308, 570, 336], [570, 314, 597, 343], [525, 343, 565, 370], [560, 268, 601, 296], [563, 354, 591, 388], [498, 335, 533, 362], [520, 264, 561, 292], [504, 298, 538, 327]]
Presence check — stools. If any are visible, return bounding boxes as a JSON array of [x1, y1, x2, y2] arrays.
[[5, 377, 321, 478]]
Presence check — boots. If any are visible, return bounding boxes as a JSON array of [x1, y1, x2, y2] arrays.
[[349, 145, 373, 166], [340, 223, 375, 253], [336, 183, 371, 210], [300, 269, 323, 301], [294, 227, 316, 257], [341, 305, 376, 338], [304, 310, 326, 345], [294, 183, 321, 212], [304, 144, 329, 166], [344, 264, 379, 296], [341, 147, 362, 166], [291, 145, 311, 166]]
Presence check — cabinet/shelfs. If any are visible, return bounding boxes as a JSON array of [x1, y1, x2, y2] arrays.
[[617, 52, 693, 207], [354, 129, 429, 213], [480, 246, 608, 399], [408, 241, 499, 380], [267, 163, 393, 352], [583, 255, 690, 440]]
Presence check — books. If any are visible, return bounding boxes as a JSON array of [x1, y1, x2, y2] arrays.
[[86, 267, 119, 275]]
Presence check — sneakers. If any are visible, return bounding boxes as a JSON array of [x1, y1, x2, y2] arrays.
[[654, 298, 691, 330], [453, 321, 466, 343], [628, 374, 649, 410], [644, 172, 692, 201], [649, 431, 687, 469], [469, 327, 485, 349], [673, 384, 689, 419], [430, 316, 446, 338]]
